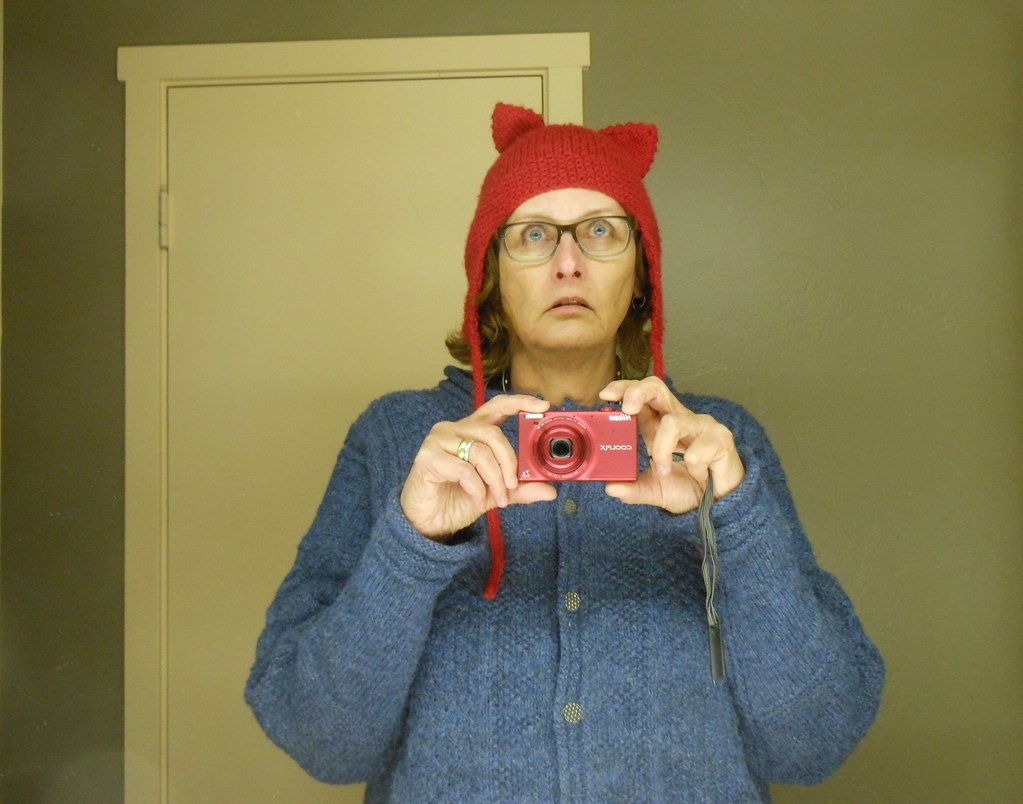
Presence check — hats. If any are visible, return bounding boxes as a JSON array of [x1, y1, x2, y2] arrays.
[[464, 101, 662, 600]]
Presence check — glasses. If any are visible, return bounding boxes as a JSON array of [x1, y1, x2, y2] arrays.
[[502, 215, 637, 264]]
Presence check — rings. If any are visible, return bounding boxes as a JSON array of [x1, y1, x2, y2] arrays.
[[457, 440, 475, 462]]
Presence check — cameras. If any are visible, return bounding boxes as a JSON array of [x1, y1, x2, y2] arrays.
[[517, 407, 638, 483]]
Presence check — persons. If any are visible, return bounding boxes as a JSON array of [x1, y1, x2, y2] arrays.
[[243, 103, 884, 804]]
